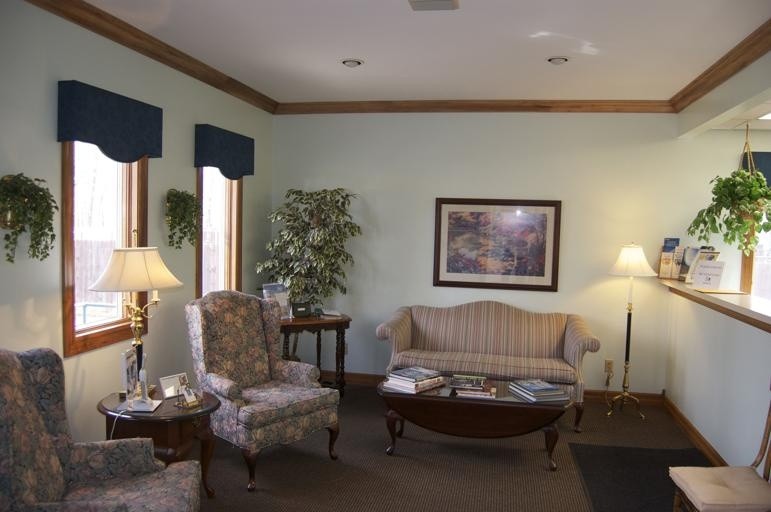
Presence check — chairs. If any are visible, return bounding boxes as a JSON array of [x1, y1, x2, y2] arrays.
[[669, 378, 771, 512]]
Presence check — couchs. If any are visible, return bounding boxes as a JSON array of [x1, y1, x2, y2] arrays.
[[184, 289, 342, 492], [375, 300, 602, 433], [1, 346, 205, 512]]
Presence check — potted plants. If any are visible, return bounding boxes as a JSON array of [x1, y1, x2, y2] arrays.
[[254, 186, 364, 318], [165, 188, 203, 250], [1, 173, 62, 264], [688, 167, 771, 258]]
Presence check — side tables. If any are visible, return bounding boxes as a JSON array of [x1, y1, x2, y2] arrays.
[[98, 385, 223, 498], [281, 311, 354, 396]]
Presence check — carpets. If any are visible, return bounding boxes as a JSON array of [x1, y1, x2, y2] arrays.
[[570, 440, 718, 512]]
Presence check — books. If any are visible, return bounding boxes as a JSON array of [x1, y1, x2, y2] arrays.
[[383, 364, 572, 403]]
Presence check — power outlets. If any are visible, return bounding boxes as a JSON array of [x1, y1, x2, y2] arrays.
[[606, 359, 614, 372]]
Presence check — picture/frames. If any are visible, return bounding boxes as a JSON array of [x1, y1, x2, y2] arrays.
[[157, 371, 191, 399], [262, 282, 292, 320], [434, 199, 562, 293], [682, 249, 723, 284], [116, 346, 140, 412]]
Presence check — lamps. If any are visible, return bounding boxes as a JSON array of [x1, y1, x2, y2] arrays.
[[86, 246, 186, 401], [606, 244, 660, 421]]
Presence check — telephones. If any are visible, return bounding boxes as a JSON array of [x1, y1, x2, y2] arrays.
[[117, 352, 163, 413]]
